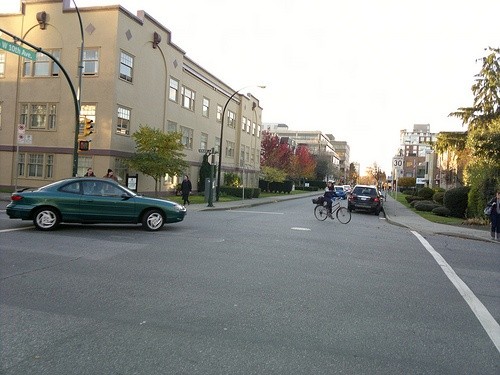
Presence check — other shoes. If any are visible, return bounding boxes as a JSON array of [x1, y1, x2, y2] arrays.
[[330, 215, 335, 219], [188, 202, 190, 206]]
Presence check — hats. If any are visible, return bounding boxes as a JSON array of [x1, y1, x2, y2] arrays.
[[107, 169, 112, 172]]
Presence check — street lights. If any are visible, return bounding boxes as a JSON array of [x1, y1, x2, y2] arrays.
[[215, 85, 267, 201]]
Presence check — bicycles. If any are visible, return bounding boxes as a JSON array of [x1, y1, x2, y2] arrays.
[[313, 192, 352, 224]]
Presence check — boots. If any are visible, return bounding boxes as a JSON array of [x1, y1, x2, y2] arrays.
[[491, 232, 495, 240], [496, 233, 500, 242]]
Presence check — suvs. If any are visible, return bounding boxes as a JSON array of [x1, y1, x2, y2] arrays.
[[346, 185, 384, 216]]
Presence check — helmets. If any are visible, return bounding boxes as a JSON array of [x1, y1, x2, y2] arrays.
[[328, 180, 336, 184]]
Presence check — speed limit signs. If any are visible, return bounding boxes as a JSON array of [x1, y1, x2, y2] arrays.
[[392, 157, 403, 169]]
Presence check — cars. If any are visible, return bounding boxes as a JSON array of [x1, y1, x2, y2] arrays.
[[6, 176, 188, 232], [333, 185, 351, 200]]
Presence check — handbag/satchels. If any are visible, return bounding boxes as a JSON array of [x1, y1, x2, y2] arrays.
[[312, 197, 324, 204], [484, 199, 494, 216]]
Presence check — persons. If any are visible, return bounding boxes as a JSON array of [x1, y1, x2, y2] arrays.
[[324, 182, 340, 219], [488, 192, 500, 240], [84, 169, 118, 195], [181, 176, 192, 206]]
